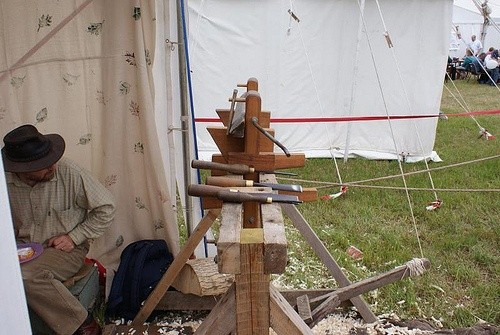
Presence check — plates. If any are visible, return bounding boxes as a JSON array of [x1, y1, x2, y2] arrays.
[[17, 244, 42, 263]]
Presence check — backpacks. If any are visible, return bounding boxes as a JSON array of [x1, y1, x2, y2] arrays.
[[104, 239, 174, 324]]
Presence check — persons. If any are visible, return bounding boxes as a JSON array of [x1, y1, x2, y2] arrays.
[[467, 34, 483, 58], [455, 47, 476, 79], [483, 49, 500, 86], [471, 53, 491, 84], [484, 47, 495, 55], [444, 56, 457, 81], [0, 124, 118, 334]]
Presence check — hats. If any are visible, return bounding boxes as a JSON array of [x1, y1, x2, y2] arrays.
[[1, 124, 66, 172]]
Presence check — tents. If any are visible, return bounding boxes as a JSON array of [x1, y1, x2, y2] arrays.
[[448, 3, 500, 63], [0, 0, 202, 335], [181, 0, 452, 164]]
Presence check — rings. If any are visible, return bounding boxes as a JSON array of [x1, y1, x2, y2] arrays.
[[61, 248, 64, 250]]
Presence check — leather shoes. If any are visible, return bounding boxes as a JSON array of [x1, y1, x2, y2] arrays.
[[77, 311, 102, 335]]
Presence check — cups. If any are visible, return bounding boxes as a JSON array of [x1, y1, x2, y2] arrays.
[[347, 246, 361, 258]]
